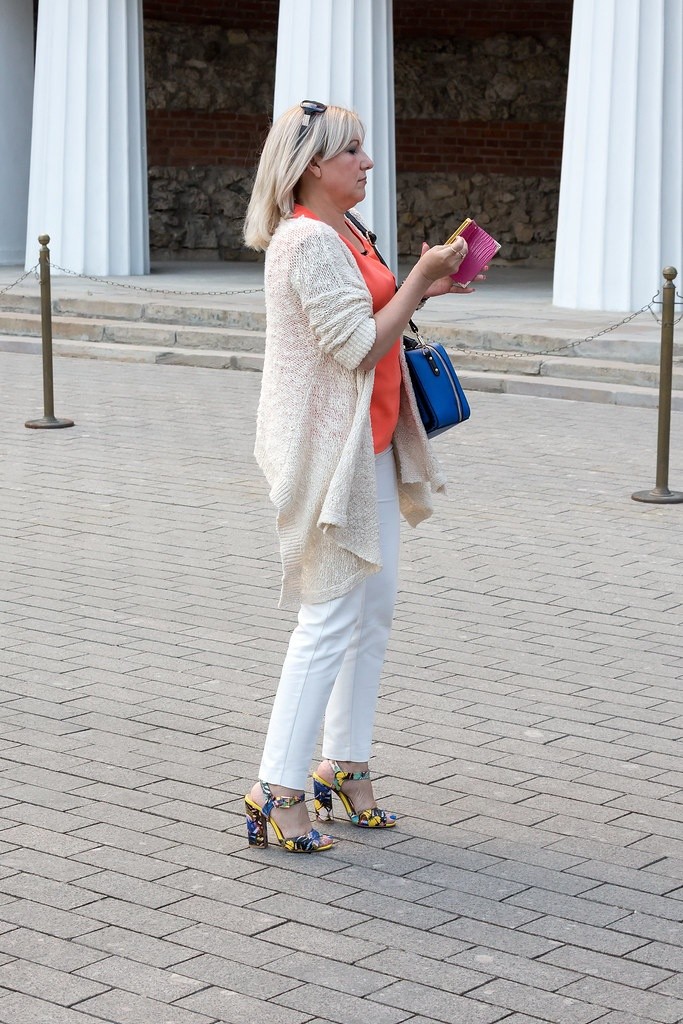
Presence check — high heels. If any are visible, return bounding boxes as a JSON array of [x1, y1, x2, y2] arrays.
[[244, 780, 333, 852], [312, 760, 397, 829]]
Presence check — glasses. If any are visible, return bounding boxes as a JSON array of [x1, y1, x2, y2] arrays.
[[294, 100, 327, 155]]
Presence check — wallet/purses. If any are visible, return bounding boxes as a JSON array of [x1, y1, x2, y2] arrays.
[[442, 218, 502, 289]]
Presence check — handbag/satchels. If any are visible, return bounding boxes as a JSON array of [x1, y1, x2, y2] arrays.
[[344, 210, 470, 440]]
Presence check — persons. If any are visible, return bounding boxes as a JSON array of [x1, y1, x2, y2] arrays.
[[243, 100, 490, 853]]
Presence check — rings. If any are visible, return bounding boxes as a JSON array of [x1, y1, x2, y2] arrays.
[[459, 251, 466, 259], [451, 247, 457, 253]]
